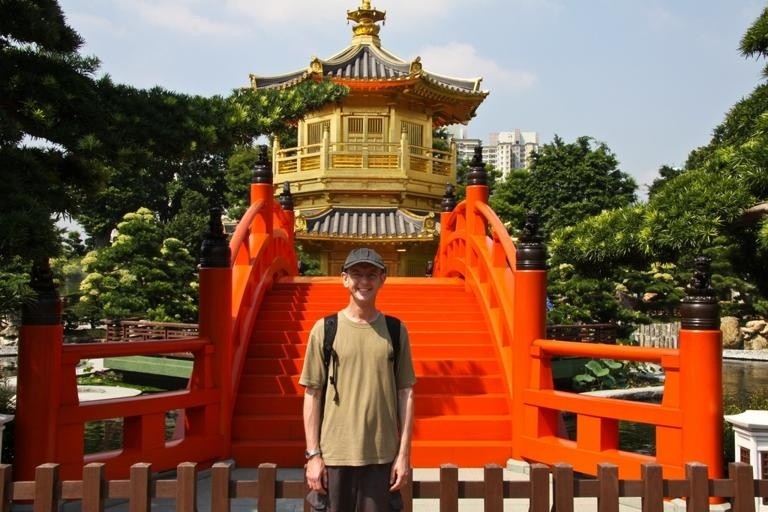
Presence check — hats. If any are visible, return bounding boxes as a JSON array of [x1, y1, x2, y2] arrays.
[[342, 247, 385, 271]]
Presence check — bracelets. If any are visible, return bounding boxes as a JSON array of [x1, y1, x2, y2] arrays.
[[304, 449, 321, 459]]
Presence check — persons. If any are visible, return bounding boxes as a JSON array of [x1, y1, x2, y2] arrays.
[[298, 247, 417, 512]]
